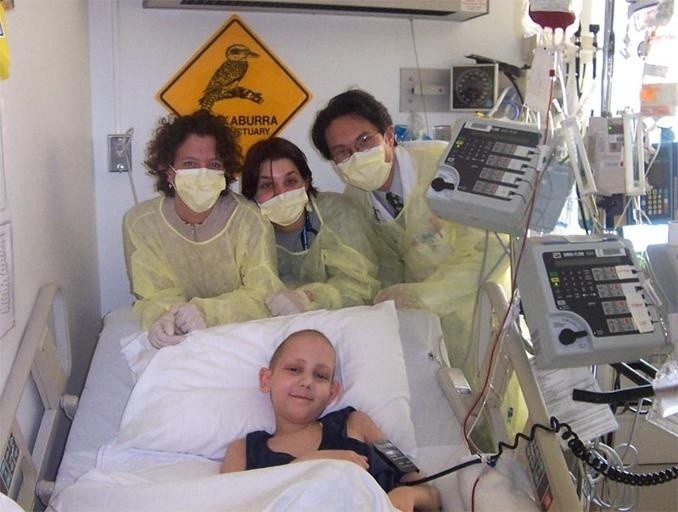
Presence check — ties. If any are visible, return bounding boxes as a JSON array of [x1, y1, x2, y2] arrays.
[[386, 193, 403, 213]]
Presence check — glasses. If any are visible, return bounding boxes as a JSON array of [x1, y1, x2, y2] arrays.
[[331, 132, 380, 162]]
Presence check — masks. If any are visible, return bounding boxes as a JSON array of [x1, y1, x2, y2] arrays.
[[167, 165, 227, 214], [331, 134, 394, 193], [254, 181, 310, 227]]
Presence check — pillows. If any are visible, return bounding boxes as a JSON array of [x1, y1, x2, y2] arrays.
[[116, 299, 421, 463]]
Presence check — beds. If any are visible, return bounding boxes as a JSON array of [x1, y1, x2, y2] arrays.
[[0, 280, 584, 511]]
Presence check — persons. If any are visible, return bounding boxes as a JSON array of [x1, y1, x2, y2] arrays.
[[119, 110, 278, 352], [219, 329, 441, 511], [310, 86, 531, 450], [239, 137, 383, 319]]
